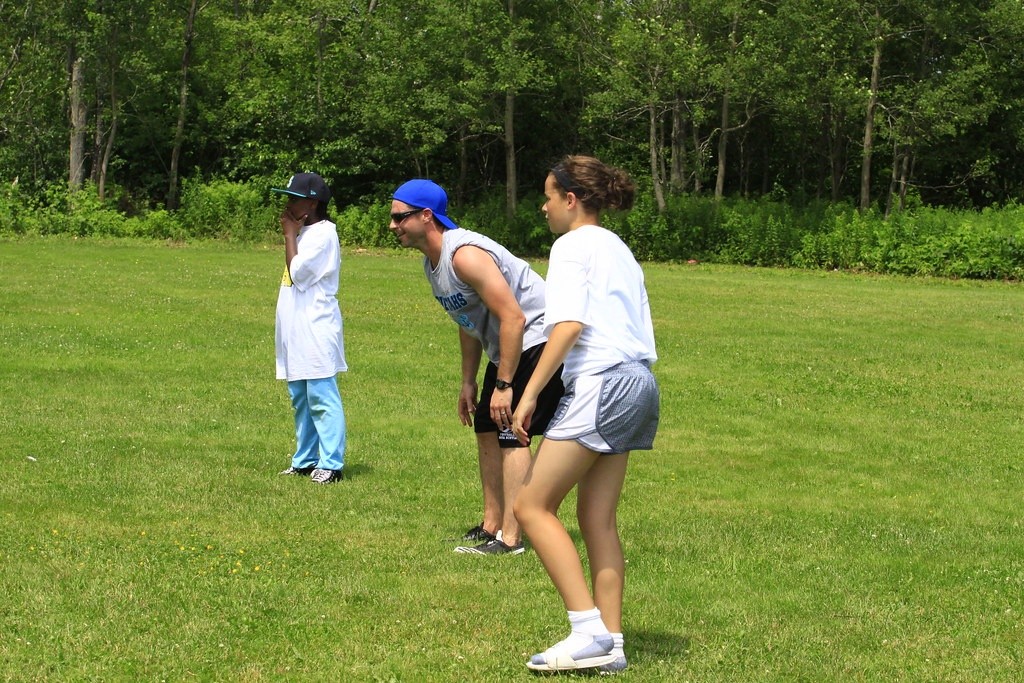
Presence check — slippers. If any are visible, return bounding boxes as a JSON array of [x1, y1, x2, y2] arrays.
[[525, 640, 617, 672]]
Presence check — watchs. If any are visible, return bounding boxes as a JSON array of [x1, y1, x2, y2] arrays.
[[492, 379, 515, 390]]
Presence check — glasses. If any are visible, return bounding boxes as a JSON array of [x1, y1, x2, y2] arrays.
[[390, 207, 426, 223]]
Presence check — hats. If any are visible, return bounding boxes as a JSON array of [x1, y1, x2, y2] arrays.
[[270, 171, 331, 203], [391, 179, 458, 229]]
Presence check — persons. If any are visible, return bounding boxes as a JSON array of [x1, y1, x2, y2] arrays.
[[388, 179, 567, 560], [508, 157, 661, 671], [274, 172, 348, 484]]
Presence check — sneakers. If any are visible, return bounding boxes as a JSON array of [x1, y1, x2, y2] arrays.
[[452, 529, 526, 555], [279, 468, 315, 477], [312, 469, 343, 484], [454, 519, 496, 543]]
